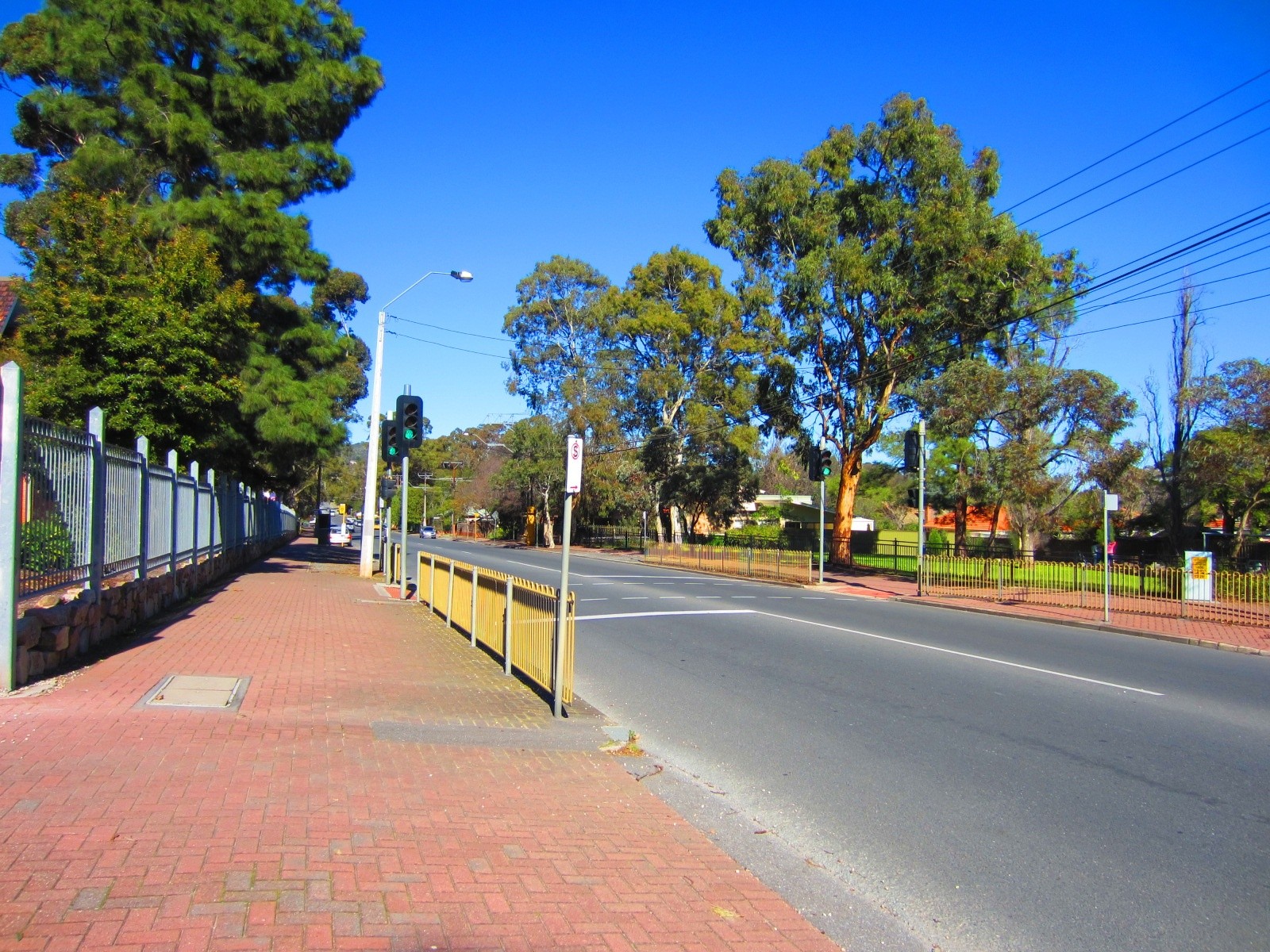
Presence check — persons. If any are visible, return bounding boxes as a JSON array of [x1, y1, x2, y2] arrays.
[[1091, 539, 1118, 566]]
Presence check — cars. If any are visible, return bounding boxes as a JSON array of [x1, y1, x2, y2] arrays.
[[1109, 524, 1234, 558], [330, 508, 363, 531], [374, 519, 380, 530], [419, 526, 437, 539], [329, 526, 352, 547]]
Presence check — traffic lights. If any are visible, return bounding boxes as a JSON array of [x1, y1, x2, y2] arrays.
[[907, 488, 920, 509], [813, 448, 832, 480], [381, 420, 403, 462], [395, 395, 423, 448]]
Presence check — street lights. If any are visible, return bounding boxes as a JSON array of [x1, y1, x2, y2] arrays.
[[359, 269, 473, 579]]
